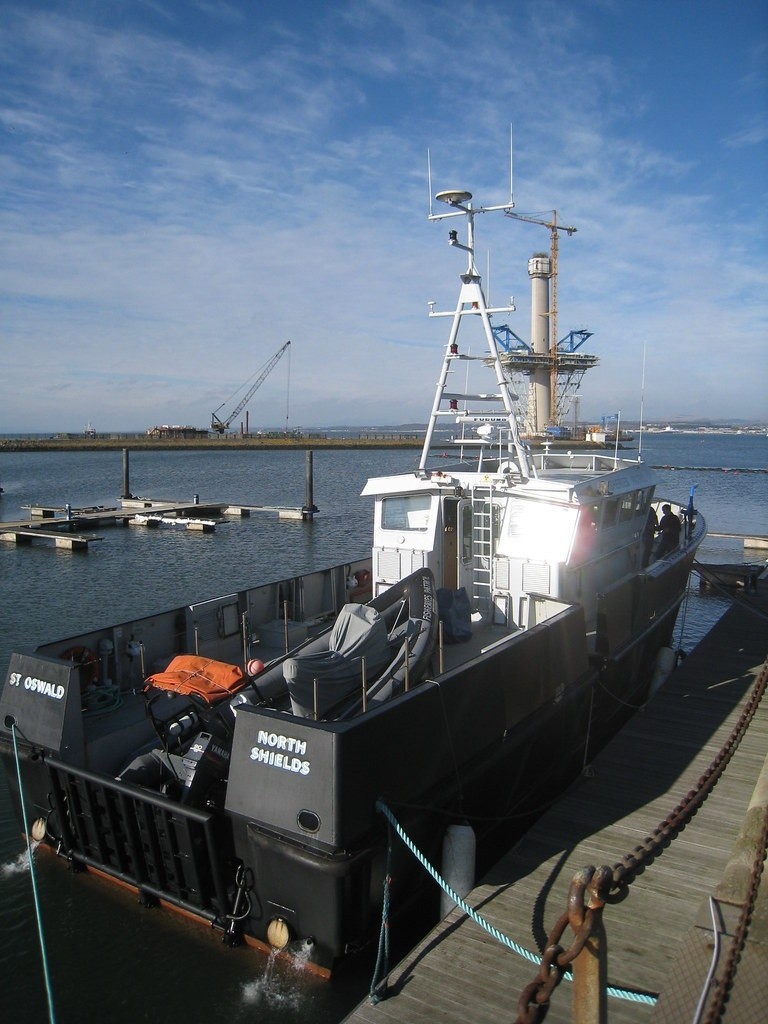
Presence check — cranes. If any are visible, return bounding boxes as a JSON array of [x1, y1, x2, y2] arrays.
[[210, 339, 291, 434]]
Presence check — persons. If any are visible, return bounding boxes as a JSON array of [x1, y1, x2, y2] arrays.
[[641, 507, 658, 569], [654, 504, 681, 562]]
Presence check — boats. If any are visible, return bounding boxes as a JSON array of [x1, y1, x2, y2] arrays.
[[1, 128, 711, 974]]
